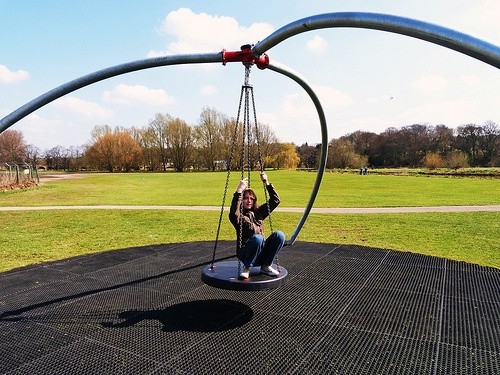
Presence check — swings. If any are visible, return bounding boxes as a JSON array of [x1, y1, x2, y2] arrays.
[[200, 67, 290, 291]]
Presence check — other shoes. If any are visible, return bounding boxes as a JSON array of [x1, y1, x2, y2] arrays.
[[259, 267, 279, 275], [240, 268, 250, 278]]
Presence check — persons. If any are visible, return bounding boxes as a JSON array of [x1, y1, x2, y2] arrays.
[[229, 172, 286, 280]]
